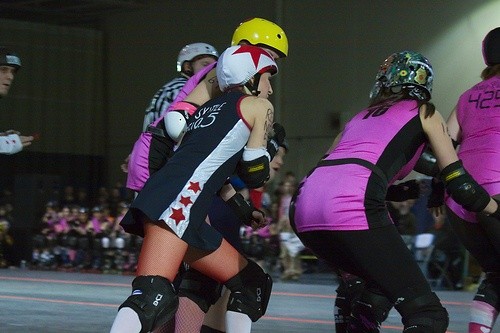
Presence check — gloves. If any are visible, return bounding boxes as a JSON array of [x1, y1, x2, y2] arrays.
[[270, 122, 286, 151]]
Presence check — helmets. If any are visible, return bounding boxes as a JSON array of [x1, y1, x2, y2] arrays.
[[376, 49, 433, 100], [231, 17, 288, 57], [177, 42, 219, 71], [216, 44, 279, 92], [482, 26, 500, 65], [0, 47, 23, 71]]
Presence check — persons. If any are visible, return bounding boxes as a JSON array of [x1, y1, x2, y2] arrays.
[[107, 43, 281, 333], [1, 43, 36, 155], [125, 43, 218, 197], [425, 26, 500, 333], [125, 16, 289, 333], [287, 49, 499, 333], [0, 167, 486, 293], [230, 142, 290, 201]]
